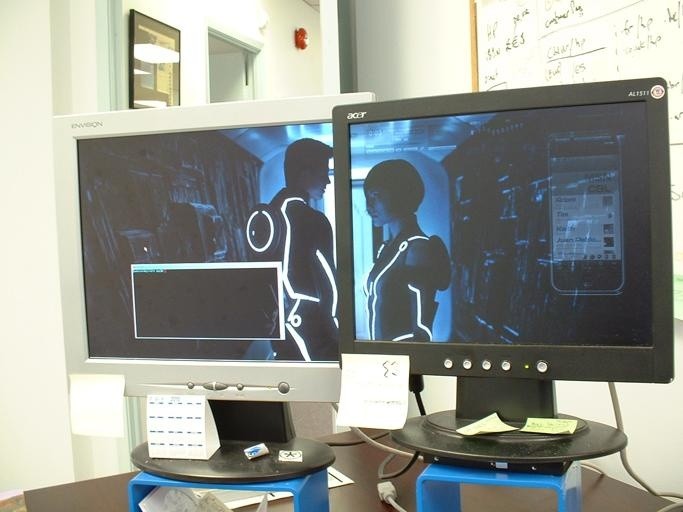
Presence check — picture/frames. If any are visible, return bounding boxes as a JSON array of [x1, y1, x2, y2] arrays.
[[128, 9, 181, 109]]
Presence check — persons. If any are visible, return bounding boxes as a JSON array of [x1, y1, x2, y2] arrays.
[[360, 158, 440, 343], [248, 137, 338, 359]]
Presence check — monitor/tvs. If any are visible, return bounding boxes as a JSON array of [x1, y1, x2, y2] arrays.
[[331, 78, 675, 463], [51, 91, 376, 485]]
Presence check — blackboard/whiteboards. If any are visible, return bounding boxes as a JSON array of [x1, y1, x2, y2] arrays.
[[470, 0, 682, 320]]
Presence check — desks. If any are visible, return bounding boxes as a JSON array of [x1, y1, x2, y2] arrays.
[[20, 429, 679, 511]]
